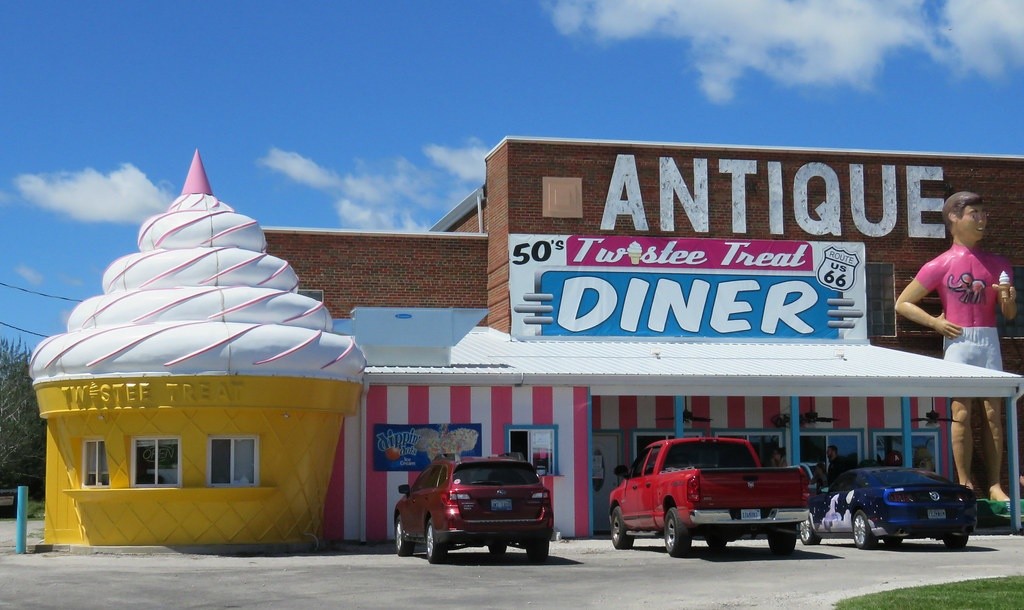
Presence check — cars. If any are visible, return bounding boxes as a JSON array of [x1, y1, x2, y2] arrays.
[[798, 467, 977, 550]]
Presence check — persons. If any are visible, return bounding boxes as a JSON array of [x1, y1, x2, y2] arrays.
[[894, 189, 1020, 505], [769, 444, 908, 498]]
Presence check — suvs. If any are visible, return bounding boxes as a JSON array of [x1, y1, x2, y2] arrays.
[[394, 452, 554, 564]]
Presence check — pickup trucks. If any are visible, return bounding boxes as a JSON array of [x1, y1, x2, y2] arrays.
[[608, 437, 811, 558]]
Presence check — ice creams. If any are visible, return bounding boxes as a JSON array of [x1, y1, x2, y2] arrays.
[[999, 270, 1010, 298]]
